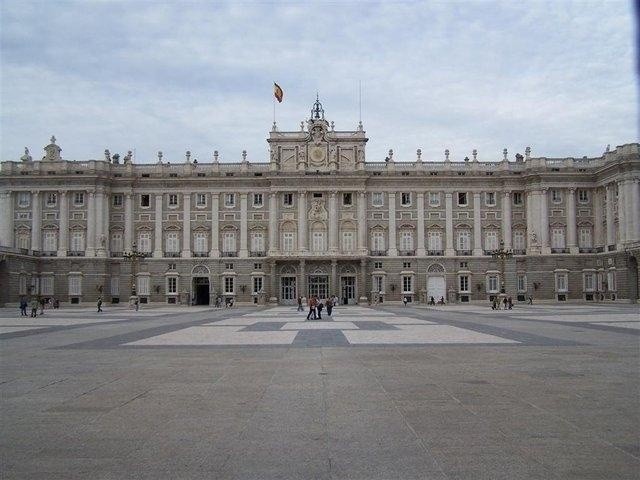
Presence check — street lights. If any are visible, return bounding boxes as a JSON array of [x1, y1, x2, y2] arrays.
[[121, 241, 153, 309], [491, 239, 514, 308]]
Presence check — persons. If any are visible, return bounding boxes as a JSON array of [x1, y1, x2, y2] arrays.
[[528, 294, 534, 306], [96, 297, 102, 313], [297, 294, 337, 320], [215, 293, 234, 308], [19, 296, 55, 317], [134, 298, 139, 311], [404, 296, 408, 307], [489, 294, 515, 312], [430, 296, 446, 305]]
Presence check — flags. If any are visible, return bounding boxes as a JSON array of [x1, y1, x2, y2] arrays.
[[274, 83, 283, 105]]
